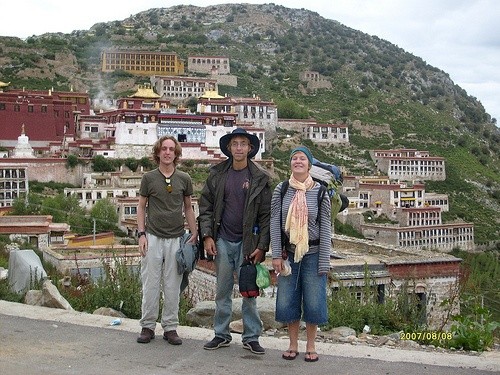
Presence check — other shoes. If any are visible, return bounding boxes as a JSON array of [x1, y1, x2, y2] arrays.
[[163, 330, 182, 345], [242, 341, 266, 354], [137, 328, 156, 343], [204, 336, 231, 350]]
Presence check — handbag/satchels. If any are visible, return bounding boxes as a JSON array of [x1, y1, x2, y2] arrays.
[[238, 258, 259, 298], [197, 218, 216, 262], [256, 262, 270, 288]]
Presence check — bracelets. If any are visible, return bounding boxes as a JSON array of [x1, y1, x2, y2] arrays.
[[137, 232, 146, 238]]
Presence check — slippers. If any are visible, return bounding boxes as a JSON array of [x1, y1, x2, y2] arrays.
[[304, 352, 319, 362], [282, 350, 299, 360]]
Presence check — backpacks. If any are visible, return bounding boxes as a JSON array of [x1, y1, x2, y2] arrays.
[[280, 157, 349, 247]]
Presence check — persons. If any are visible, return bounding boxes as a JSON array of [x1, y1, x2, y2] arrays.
[[199, 128, 273, 354], [137, 136, 198, 346], [270, 147, 331, 362]]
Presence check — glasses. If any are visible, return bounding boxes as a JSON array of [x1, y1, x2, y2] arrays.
[[229, 141, 251, 147], [165, 177, 173, 194]]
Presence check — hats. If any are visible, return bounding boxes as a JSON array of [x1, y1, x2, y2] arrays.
[[288, 147, 313, 163], [219, 128, 260, 159]]
